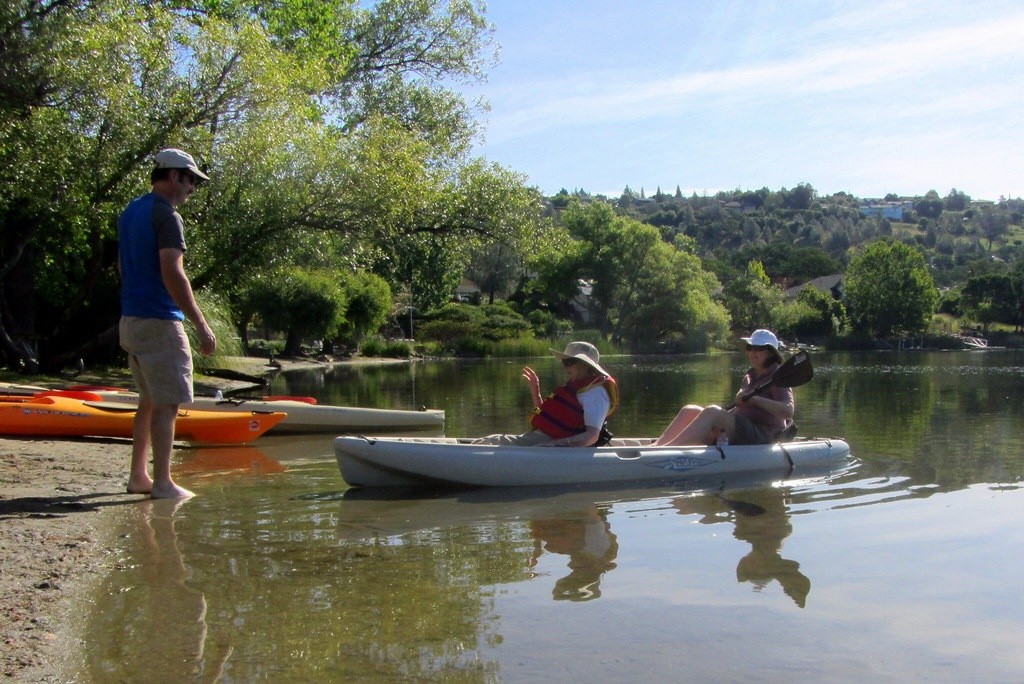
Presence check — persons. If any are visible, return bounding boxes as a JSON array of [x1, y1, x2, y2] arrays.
[[643, 328, 794, 445], [118, 147, 217, 497], [469, 341, 618, 448], [671, 484, 792, 585], [530, 484, 618, 601], [133, 497, 235, 684]]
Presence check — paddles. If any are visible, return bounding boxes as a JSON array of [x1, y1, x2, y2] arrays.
[[724, 350, 814, 411]]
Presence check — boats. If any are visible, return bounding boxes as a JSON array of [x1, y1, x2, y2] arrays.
[[0, 382, 445, 447], [333, 433, 851, 493]]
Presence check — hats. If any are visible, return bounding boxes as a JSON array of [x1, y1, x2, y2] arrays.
[[152, 148, 211, 181], [550, 341, 612, 377], [741, 329, 779, 352]]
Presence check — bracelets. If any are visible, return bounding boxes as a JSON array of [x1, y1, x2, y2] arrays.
[[566, 438, 570, 446]]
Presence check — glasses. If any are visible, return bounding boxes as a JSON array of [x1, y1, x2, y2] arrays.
[[745, 343, 770, 351], [561, 357, 578, 368], [183, 172, 200, 188]]
